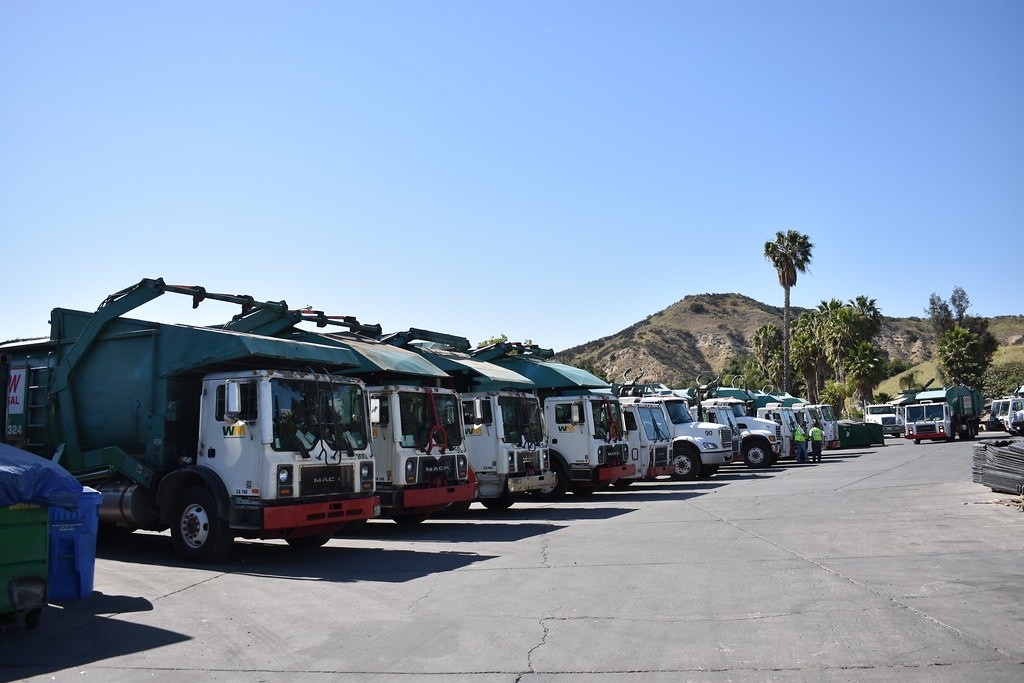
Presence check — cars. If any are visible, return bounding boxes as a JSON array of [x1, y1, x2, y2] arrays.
[[1012, 409, 1024, 436]]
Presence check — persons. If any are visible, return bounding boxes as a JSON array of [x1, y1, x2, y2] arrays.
[[795, 423, 810, 464], [809, 423, 824, 463]]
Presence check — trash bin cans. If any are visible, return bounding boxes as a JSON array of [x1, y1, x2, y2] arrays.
[[47, 485, 104, 603], [838, 422, 885, 448], [0, 501, 49, 626]]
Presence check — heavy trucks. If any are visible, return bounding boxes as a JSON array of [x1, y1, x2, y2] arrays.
[[905, 385, 985, 444], [0, 278, 840, 566]]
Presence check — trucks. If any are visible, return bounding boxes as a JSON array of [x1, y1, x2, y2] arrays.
[[989, 396, 1024, 429], [865, 386, 947, 437]]
